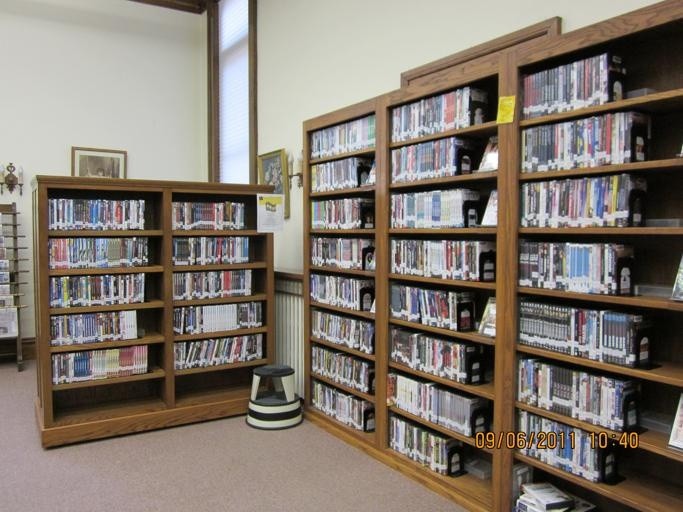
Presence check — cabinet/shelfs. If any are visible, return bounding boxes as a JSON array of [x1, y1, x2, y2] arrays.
[[0, 202, 28, 371], [302, 101, 380, 465], [502, 0, 683, 512], [168, 182, 276, 428], [380, 50, 504, 511], [29, 175, 170, 448]]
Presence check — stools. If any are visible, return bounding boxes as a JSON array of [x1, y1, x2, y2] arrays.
[[248, 364, 303, 429]]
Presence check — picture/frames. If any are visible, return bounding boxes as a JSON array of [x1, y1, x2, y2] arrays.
[[72, 147, 127, 179], [257, 148, 290, 220]]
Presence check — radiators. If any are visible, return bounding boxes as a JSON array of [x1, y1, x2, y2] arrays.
[[273, 271, 304, 405]]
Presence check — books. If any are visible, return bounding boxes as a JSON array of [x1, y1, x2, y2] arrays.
[[311, 115, 375, 434], [388, 370, 490, 437], [520, 54, 647, 172], [517, 174, 647, 296], [670, 252, 683, 303], [171, 201, 263, 370], [391, 326, 484, 384], [390, 237, 496, 282], [0, 213, 19, 338], [48, 198, 148, 386], [389, 413, 493, 478], [391, 87, 487, 140], [391, 136, 498, 183], [390, 281, 497, 338], [390, 188, 497, 229], [516, 301, 651, 432], [513, 465, 597, 512], [668, 390, 683, 451], [518, 411, 617, 484]]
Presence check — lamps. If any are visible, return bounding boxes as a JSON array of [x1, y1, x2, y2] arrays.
[[0, 161, 24, 196], [285, 155, 303, 187]]
